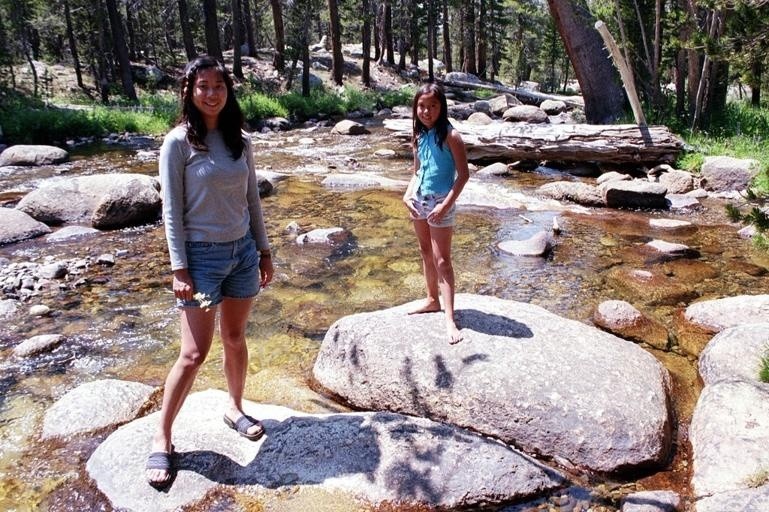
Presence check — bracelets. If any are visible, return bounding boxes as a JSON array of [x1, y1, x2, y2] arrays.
[[260, 253, 272, 259]]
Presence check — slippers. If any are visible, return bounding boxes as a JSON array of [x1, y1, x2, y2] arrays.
[[223, 415, 264, 440], [146, 444, 174, 487]]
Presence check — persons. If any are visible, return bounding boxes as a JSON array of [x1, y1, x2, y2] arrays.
[[402, 83, 470, 345], [145, 57, 274, 486]]
